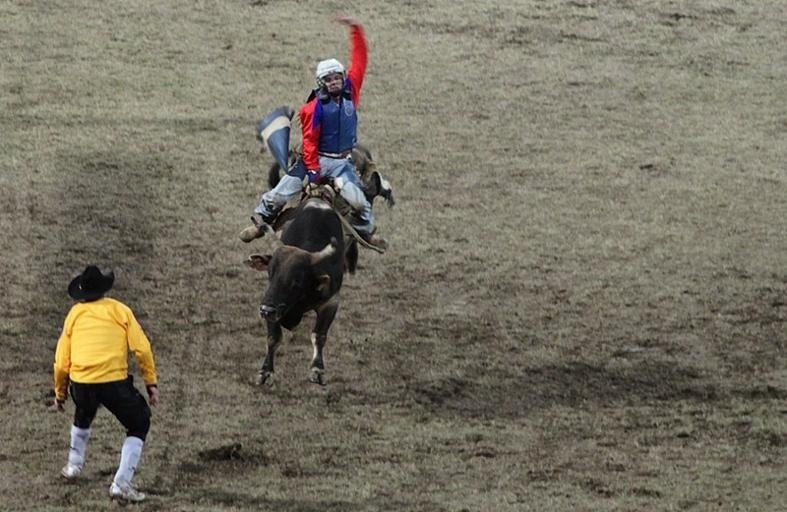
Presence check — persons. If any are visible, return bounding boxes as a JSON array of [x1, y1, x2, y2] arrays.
[[50, 262, 163, 504], [236, 11, 390, 254]]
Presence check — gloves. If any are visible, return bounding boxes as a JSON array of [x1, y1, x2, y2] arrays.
[[304, 170, 320, 197]]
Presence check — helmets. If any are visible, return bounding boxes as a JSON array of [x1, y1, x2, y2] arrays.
[[314, 58, 348, 99]]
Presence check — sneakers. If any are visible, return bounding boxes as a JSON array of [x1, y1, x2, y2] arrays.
[[238, 214, 276, 243], [359, 225, 389, 255], [109, 478, 147, 503], [60, 463, 82, 481]]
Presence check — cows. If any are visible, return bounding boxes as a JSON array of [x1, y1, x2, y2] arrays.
[[240, 144, 397, 388]]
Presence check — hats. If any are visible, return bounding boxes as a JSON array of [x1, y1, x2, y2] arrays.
[[67, 264, 116, 301]]
